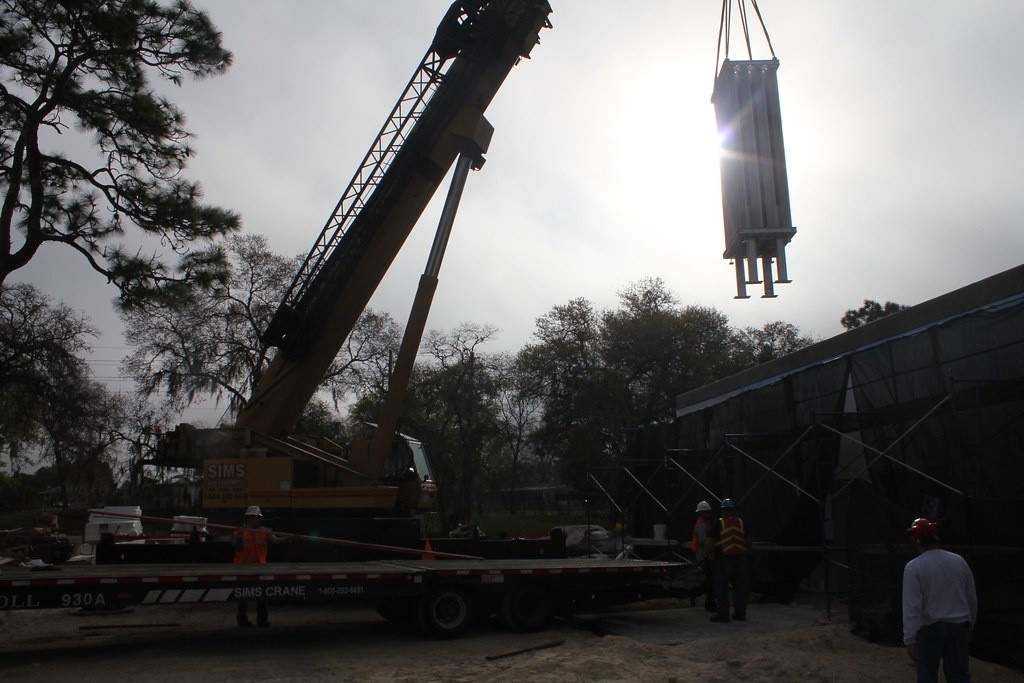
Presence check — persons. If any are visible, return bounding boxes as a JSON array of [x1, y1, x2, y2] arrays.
[[710, 498, 752, 621], [903, 518, 978, 683], [232, 506, 291, 626], [688, 501, 717, 612]]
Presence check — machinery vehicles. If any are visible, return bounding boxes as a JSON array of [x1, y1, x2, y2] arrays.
[[70, 0, 796, 618]]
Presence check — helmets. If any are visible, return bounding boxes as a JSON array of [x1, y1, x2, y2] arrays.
[[695, 501, 712, 512], [721, 498, 735, 508], [245, 506, 262, 517], [911, 518, 939, 542]]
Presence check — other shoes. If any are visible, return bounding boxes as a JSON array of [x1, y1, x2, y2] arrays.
[[711, 614, 729, 622], [238, 621, 252, 627], [732, 610, 746, 621], [705, 600, 718, 612], [257, 619, 270, 627], [686, 588, 696, 607]]
